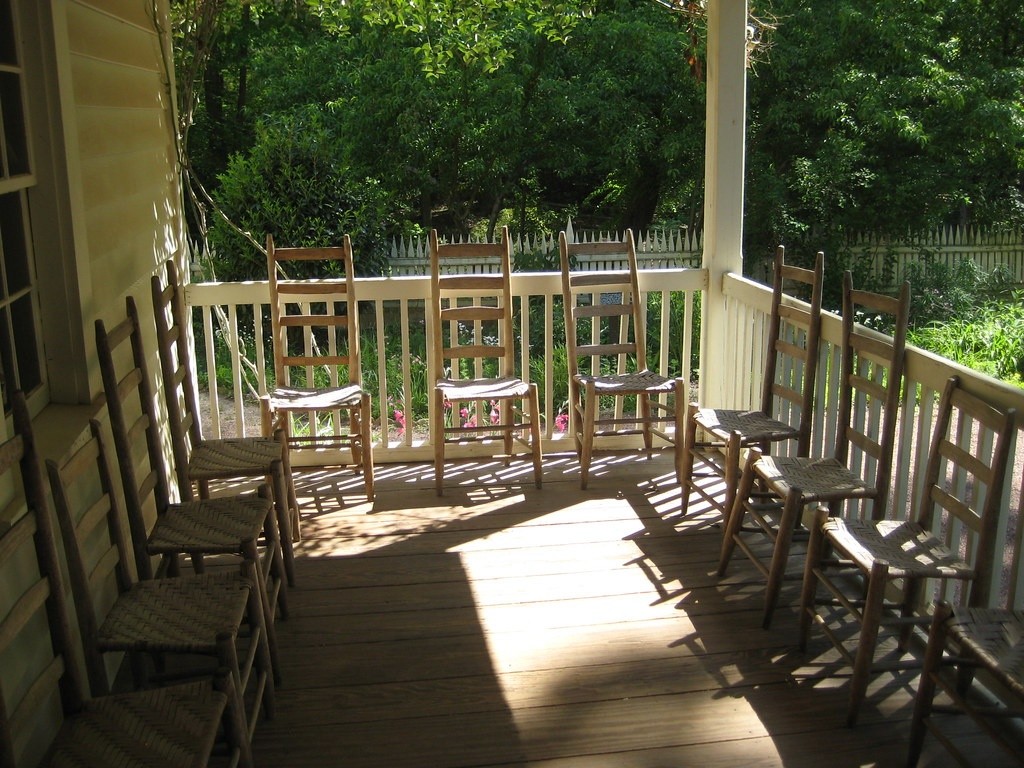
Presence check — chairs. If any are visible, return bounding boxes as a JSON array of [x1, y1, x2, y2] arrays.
[[0, 389, 253, 768], [430, 226, 542, 498], [715, 269, 912, 629], [260, 232, 374, 502], [680, 244, 825, 554], [93, 297, 289, 682], [902, 459, 1024, 768], [43, 418, 276, 768], [150, 260, 301, 586], [558, 229, 684, 489], [795, 374, 1018, 719]]
